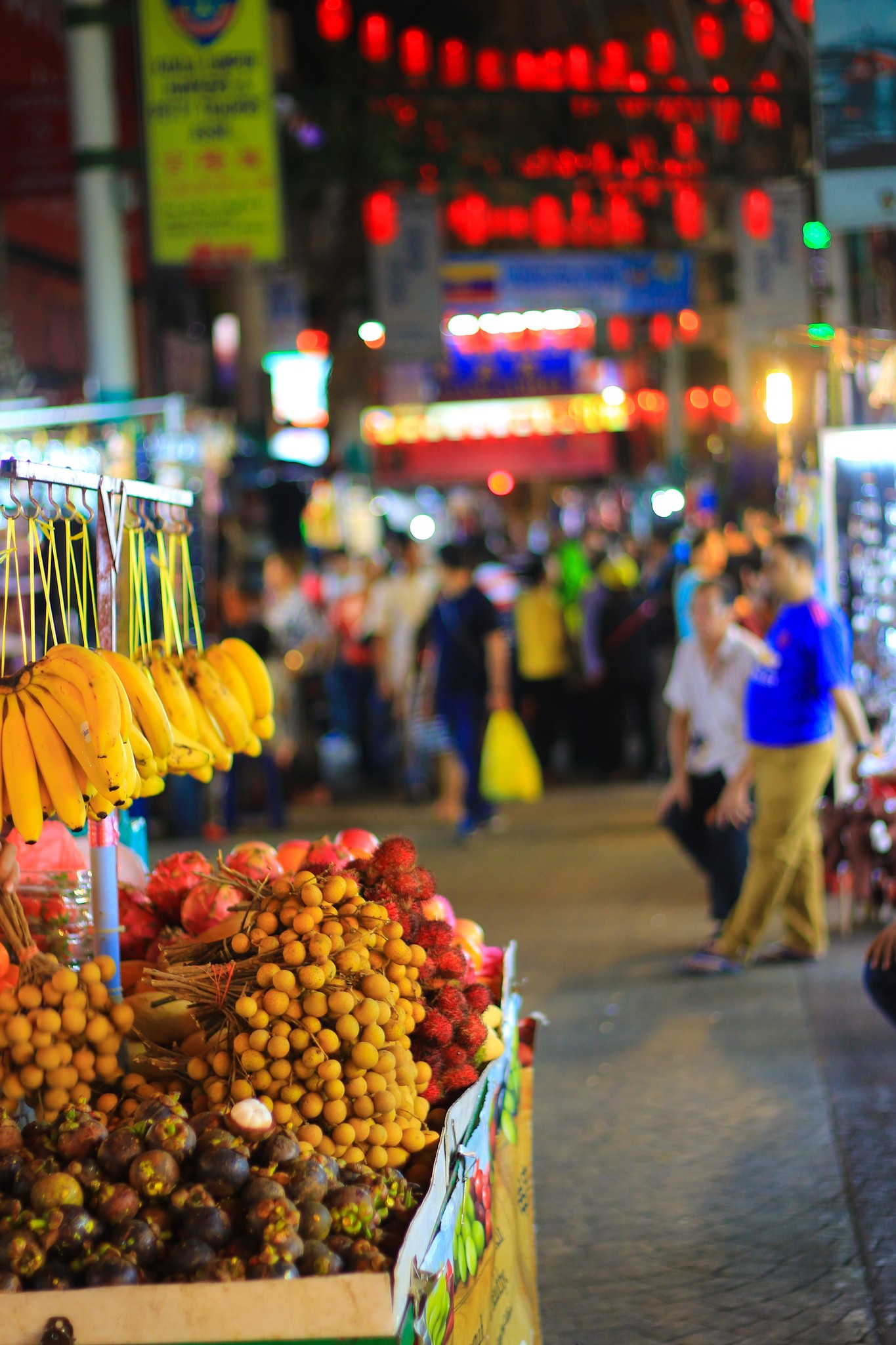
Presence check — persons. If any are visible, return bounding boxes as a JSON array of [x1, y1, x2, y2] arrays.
[[674, 537, 887, 977], [201, 506, 787, 845], [650, 580, 768, 938], [0, 835, 20, 894], [863, 918, 896, 1028]]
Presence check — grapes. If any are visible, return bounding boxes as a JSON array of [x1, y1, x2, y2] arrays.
[[454, 1160, 492, 1283], [425, 1259, 453, 1345]]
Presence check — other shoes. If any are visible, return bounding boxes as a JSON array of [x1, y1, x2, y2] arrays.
[[679, 952, 744, 976], [696, 930, 723, 950], [755, 942, 806, 964]]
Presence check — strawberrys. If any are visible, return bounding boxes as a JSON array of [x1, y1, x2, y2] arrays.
[[15, 889, 89, 964]]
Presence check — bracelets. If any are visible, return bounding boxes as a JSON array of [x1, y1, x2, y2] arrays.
[[856, 740, 876, 755]]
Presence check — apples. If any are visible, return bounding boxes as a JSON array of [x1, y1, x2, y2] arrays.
[[230, 828, 486, 972]]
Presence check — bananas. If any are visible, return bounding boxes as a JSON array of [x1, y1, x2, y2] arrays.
[[1, 636, 273, 844]]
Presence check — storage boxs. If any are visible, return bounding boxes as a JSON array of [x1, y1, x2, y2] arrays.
[[0, 938, 520, 1345]]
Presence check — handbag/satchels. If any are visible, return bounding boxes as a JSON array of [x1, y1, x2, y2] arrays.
[[475, 707, 545, 805]]
